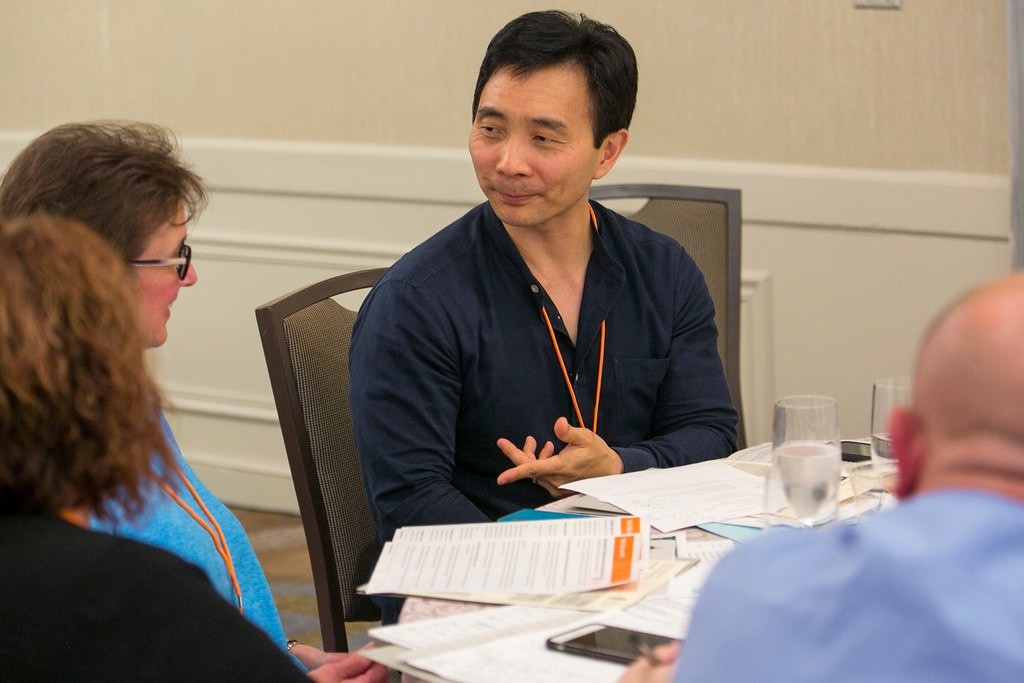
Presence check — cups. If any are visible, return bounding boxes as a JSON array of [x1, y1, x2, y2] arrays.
[[870, 378, 915, 507], [770, 396, 842, 525]]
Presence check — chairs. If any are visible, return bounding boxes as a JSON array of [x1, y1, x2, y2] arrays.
[[254, 266, 394, 654], [587, 182, 747, 451]]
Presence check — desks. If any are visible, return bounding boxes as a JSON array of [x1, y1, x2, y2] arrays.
[[397, 435, 903, 683]]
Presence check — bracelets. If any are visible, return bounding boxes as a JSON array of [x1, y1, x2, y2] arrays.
[[287, 638, 299, 649]]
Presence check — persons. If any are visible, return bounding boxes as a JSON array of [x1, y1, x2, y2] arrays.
[[338, 8, 738, 626], [0, 211, 319, 682], [0, 118, 681, 682], [675, 271, 1022, 681]]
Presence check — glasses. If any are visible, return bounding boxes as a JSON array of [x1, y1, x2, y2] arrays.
[[127, 244, 192, 280]]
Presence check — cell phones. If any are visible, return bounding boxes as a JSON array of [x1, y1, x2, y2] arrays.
[[546, 623, 683, 664], [840, 441, 872, 463]]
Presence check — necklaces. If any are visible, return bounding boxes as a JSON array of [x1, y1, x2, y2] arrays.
[[546, 202, 609, 431], [152, 454, 243, 615]]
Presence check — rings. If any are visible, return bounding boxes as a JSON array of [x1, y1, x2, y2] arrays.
[[532, 476, 538, 484]]
